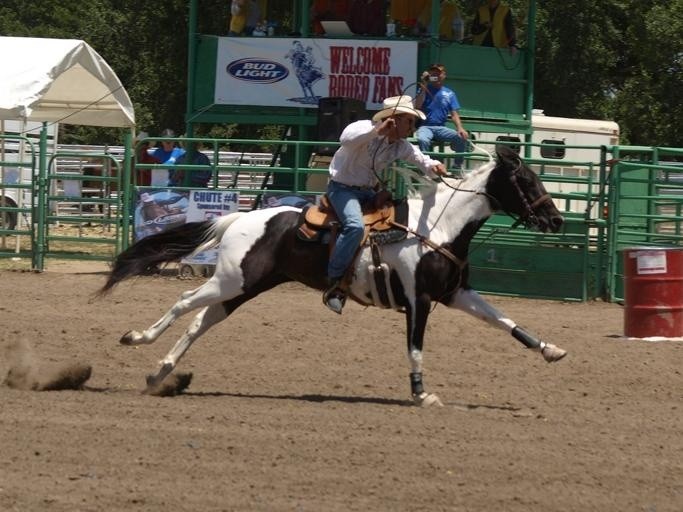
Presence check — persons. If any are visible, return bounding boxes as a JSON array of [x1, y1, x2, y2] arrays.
[[173, 131, 211, 187], [322, 95, 447, 314], [140, 192, 190, 232], [285, 41, 322, 97], [130, 130, 161, 186], [228, 0, 517, 55], [415, 64, 468, 178], [151, 128, 186, 186]]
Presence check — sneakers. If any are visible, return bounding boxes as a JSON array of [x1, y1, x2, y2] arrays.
[[325, 286, 342, 312]]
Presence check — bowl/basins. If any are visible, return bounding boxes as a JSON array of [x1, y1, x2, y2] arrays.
[[154, 211, 186, 230]]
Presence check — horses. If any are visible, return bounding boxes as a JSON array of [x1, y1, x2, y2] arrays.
[[90, 144, 567, 410], [140, 195, 186, 233], [82, 153, 134, 226]]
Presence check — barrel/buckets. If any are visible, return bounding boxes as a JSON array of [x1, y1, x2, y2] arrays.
[[623, 246, 683, 337]]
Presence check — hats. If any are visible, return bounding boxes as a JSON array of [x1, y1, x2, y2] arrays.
[[132, 132, 157, 147], [161, 129, 175, 138], [179, 134, 203, 150], [430, 62, 443, 71], [372, 94, 427, 121]]
